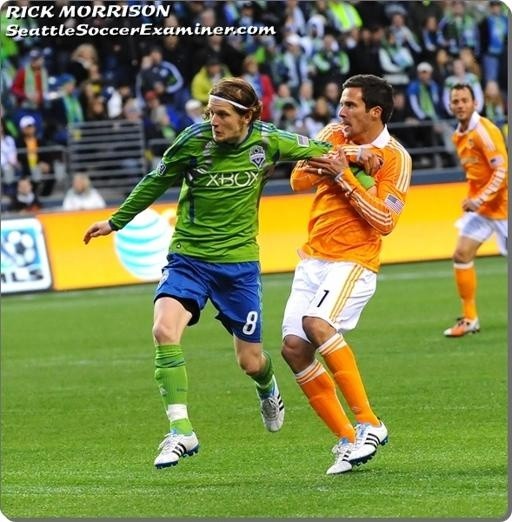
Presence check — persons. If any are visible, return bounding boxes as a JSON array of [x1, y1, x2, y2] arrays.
[[0, 1, 508, 214], [83, 77, 382, 473], [440, 82, 509, 337], [279, 74, 412, 475]]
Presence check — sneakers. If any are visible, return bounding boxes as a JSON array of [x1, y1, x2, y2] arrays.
[[256, 374, 285, 432], [154, 432, 199, 469], [443, 316, 480, 337], [325, 417, 389, 475]]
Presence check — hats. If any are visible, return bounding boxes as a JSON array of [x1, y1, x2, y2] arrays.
[[19, 115, 36, 127], [29, 47, 42, 58], [417, 61, 432, 72]]
